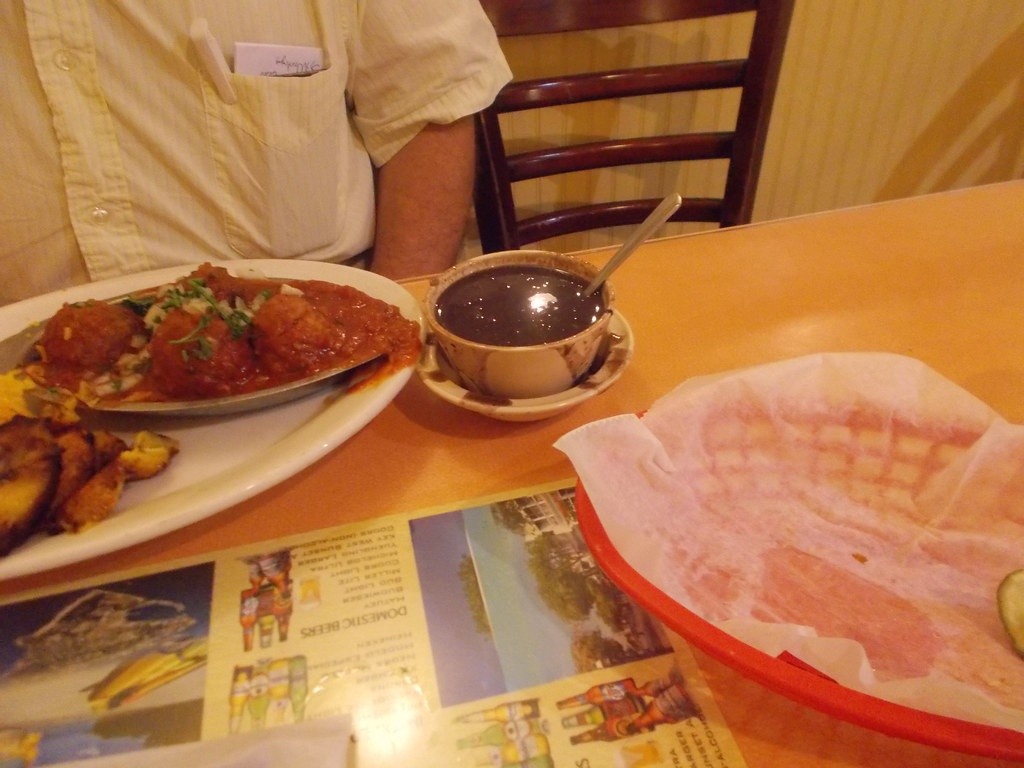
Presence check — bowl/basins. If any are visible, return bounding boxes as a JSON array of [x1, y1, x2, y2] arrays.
[[424, 248, 615, 399]]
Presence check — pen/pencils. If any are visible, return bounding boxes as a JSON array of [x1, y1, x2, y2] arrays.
[[189, 17, 237, 105]]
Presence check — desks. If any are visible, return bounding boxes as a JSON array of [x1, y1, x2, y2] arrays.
[[0, 174, 1024, 768]]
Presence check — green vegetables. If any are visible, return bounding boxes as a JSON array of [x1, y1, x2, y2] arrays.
[[124, 280, 253, 357]]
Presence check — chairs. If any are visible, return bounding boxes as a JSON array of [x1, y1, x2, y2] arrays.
[[470, 0, 799, 255]]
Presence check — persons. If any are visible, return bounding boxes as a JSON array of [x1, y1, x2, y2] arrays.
[[1, 1, 478, 308]]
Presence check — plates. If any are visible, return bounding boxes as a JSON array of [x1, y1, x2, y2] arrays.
[[25, 278, 386, 417], [415, 306, 635, 422], [0, 258, 425, 582]]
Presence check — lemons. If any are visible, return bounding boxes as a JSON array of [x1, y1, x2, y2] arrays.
[[997, 569, 1024, 658]]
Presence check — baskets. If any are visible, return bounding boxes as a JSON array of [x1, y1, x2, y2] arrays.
[[575, 402, 1024, 763]]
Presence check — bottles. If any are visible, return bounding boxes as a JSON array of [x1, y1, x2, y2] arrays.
[[240, 548, 293, 650], [227, 656, 306, 736], [555, 673, 698, 746], [453, 698, 554, 768]]
